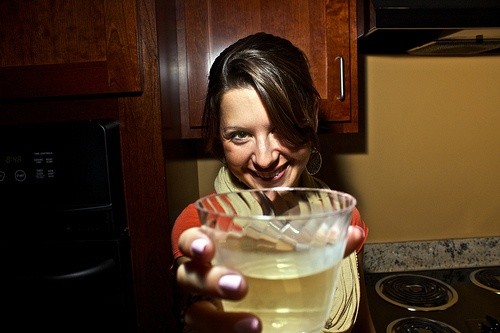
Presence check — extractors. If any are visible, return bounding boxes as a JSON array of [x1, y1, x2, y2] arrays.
[[357, 1, 500, 58]]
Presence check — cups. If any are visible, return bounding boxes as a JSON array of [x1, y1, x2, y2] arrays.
[[194, 188, 358, 333]]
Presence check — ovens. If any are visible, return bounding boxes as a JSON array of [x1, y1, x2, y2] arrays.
[[0, 119, 136, 333]]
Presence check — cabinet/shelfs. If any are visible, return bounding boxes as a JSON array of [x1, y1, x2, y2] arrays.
[[156, 0, 362, 140], [0, 0, 175, 333]]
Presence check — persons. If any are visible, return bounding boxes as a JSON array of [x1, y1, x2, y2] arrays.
[[170, 32, 378, 333]]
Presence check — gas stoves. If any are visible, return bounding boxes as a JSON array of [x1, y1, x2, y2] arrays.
[[362, 264, 500, 333]]
